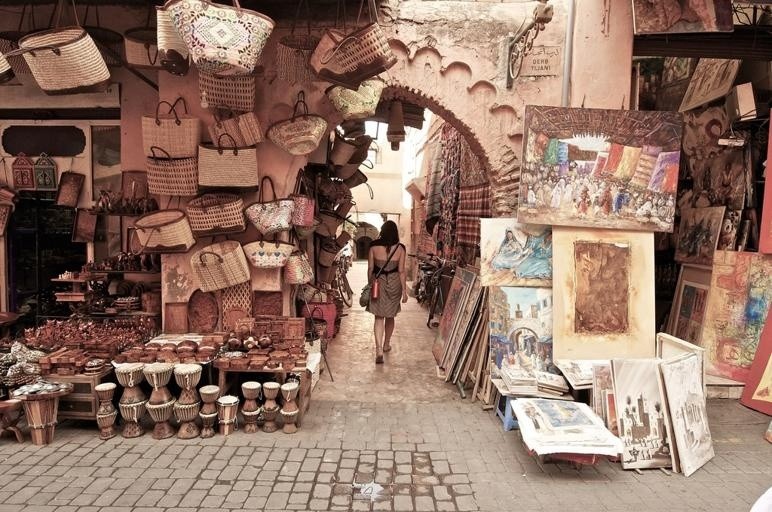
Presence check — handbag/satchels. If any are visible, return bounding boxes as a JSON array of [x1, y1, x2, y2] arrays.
[[18, 26, 113, 96], [208, 111, 265, 148], [318, 247, 338, 268], [145, 156, 198, 196], [26, 26, 126, 68], [133, 208, 197, 254], [328, 134, 358, 167], [164, 0, 277, 77], [155, 5, 195, 76], [315, 177, 354, 219], [332, 22, 398, 84], [315, 210, 344, 238], [335, 162, 359, 179], [243, 198, 295, 237], [0, 51, 24, 86], [305, 317, 328, 390], [323, 77, 386, 121], [359, 284, 372, 307], [308, 27, 362, 91], [288, 193, 315, 227], [198, 69, 256, 112], [340, 119, 366, 138], [123, 26, 164, 71], [185, 192, 248, 238], [283, 249, 315, 285], [198, 144, 259, 193], [141, 114, 202, 159], [243, 240, 296, 269], [317, 262, 338, 285], [265, 113, 329, 156], [343, 168, 368, 189], [190, 240, 251, 292], [277, 33, 328, 82], [350, 135, 373, 162], [296, 282, 341, 304], [1, 30, 32, 74]]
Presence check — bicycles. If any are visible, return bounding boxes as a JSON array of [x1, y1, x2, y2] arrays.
[[410, 253, 455, 329], [336, 256, 355, 307]]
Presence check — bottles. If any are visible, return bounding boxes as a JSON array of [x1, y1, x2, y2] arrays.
[[372, 279, 380, 298]]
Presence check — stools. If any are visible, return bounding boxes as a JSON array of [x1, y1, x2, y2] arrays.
[[0, 399, 26, 443]]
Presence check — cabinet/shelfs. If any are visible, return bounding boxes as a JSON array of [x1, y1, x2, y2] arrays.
[[38, 366, 114, 420]]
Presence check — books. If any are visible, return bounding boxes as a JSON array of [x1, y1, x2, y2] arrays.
[[499, 365, 570, 398]]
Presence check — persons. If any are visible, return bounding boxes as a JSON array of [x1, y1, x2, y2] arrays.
[[527, 171, 674, 230], [367, 220, 408, 364], [493, 228, 525, 269]]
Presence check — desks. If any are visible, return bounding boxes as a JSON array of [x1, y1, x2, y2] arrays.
[[110, 330, 231, 384], [214, 350, 317, 428]]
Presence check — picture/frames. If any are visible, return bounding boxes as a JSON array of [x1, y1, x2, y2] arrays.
[[657, 352, 697, 477], [657, 333, 707, 399], [589, 358, 672, 470], [429, 262, 499, 408], [672, 280, 710, 350], [660, 352, 716, 476]]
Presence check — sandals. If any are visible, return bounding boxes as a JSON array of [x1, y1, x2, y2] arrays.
[[375, 346, 384, 364], [383, 346, 391, 352]]
